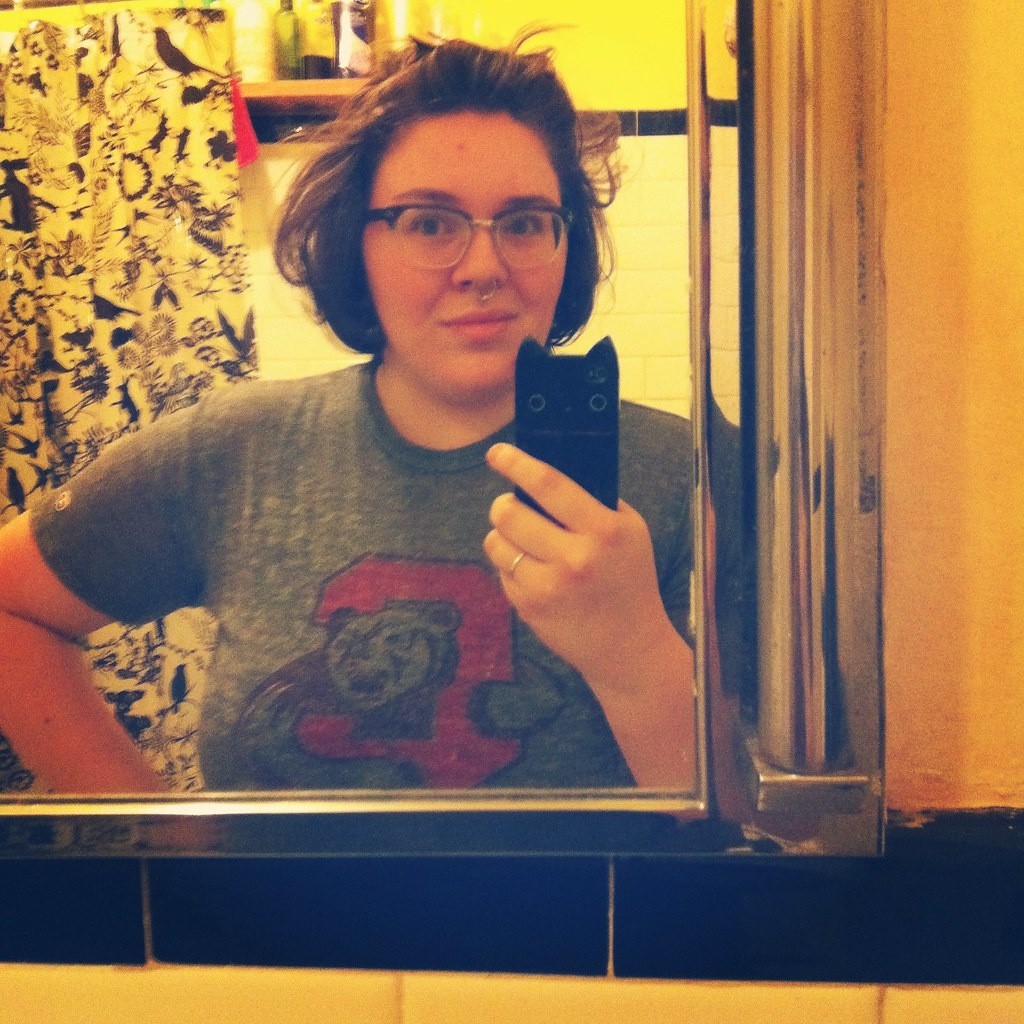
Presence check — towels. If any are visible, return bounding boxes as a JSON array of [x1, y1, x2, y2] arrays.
[[231, 69, 261, 169]]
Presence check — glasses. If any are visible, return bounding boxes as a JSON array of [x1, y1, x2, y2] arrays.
[[367, 202, 568, 271]]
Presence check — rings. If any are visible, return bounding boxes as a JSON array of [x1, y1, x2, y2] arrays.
[[505, 553, 526, 577]]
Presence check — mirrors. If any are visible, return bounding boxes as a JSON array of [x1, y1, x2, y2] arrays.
[[0, 0, 886, 861]]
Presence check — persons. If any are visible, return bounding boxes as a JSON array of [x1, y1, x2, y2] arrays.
[[1, 33, 702, 790]]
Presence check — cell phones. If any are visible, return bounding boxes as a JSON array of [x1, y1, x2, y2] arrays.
[[515, 335, 619, 528]]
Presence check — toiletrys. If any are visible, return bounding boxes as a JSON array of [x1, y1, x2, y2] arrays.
[[273, 0, 371, 79]]
